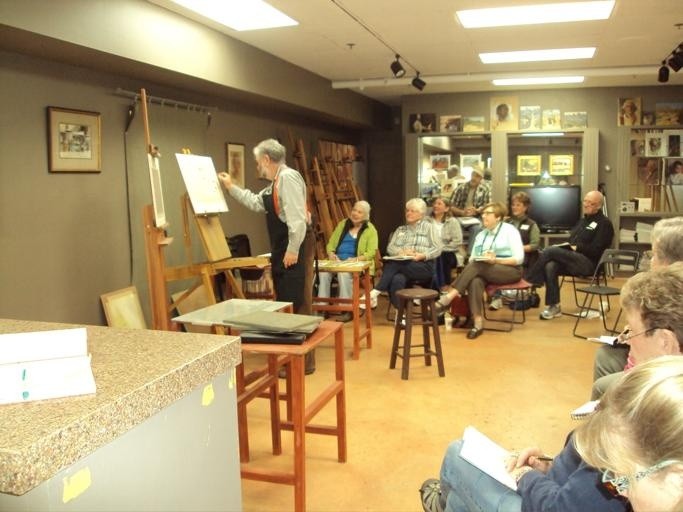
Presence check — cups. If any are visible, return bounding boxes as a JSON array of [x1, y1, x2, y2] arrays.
[[444, 317, 452, 329]]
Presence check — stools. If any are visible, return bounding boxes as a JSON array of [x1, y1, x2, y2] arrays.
[[389, 288, 446, 380]]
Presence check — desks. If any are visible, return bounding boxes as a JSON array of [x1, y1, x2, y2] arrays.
[[235, 318, 347, 511], [255, 256, 373, 363], [0, 316, 245, 511]]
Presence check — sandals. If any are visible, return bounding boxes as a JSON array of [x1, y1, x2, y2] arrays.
[[467, 327, 483, 339], [427, 301, 451, 314]]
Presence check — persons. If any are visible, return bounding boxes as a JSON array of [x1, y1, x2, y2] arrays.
[[501, 190, 615, 320], [419, 265, 683, 511], [358, 198, 442, 326], [668, 161, 683, 184], [427, 196, 464, 293], [428, 165, 459, 197], [426, 204, 525, 338], [317, 200, 378, 323], [619, 100, 638, 127], [487, 191, 540, 310], [217, 138, 312, 314], [450, 168, 491, 260], [571, 354, 683, 512], [592, 216, 683, 408]]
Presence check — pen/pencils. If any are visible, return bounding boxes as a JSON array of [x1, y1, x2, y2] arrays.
[[536, 456, 553, 461], [21, 368, 29, 402], [613, 330, 629, 342]]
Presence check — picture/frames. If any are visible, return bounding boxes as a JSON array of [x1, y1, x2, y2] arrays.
[[223, 141, 247, 188], [42, 103, 104, 177], [614, 94, 683, 188], [97, 284, 146, 330], [406, 95, 588, 178]]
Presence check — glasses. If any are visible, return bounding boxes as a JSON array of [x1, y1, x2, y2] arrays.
[[480, 211, 496, 216], [616, 324, 660, 345], [596, 459, 683, 500]]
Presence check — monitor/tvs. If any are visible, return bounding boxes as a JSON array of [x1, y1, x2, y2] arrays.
[[509, 185, 580, 234]]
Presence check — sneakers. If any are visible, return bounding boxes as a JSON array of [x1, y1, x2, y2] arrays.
[[418, 478, 445, 512], [501, 289, 529, 301], [316, 310, 330, 320], [359, 293, 378, 311], [335, 311, 353, 323], [279, 369, 315, 378], [395, 308, 406, 325], [488, 299, 504, 310], [539, 304, 563, 320]]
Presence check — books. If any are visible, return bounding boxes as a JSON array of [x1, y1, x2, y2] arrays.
[[222, 310, 325, 344], [458, 425, 519, 492], [0, 326, 96, 405], [587, 333, 618, 347], [383, 256, 415, 261], [571, 400, 600, 416], [551, 242, 575, 250], [619, 220, 654, 242]]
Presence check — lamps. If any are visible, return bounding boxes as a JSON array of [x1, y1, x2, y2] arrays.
[[389, 53, 425, 93], [655, 44, 683, 84]]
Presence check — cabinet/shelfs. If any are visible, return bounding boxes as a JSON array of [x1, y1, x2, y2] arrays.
[[613, 210, 681, 274]]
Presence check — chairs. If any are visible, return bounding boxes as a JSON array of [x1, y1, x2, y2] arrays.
[[558, 266, 611, 320], [481, 278, 532, 332], [574, 249, 639, 340], [386, 260, 438, 323], [213, 232, 272, 303]]
